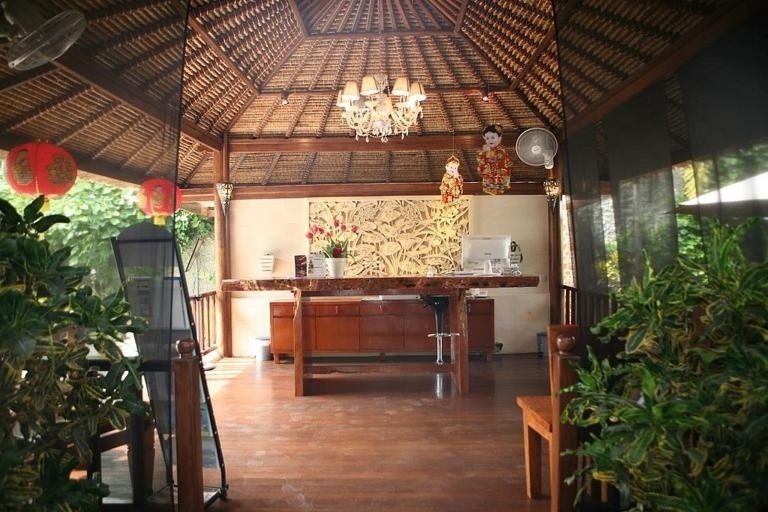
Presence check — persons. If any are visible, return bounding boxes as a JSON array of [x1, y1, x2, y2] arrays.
[[438, 155, 464, 205], [476, 123, 513, 196]]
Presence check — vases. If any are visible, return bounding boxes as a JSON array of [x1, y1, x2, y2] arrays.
[[324, 258, 346, 279]]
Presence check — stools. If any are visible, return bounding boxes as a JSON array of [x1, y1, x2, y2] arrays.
[[419, 294, 460, 366]]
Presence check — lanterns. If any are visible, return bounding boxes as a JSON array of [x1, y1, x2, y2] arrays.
[[134, 179, 183, 225], [2, 139, 79, 213]]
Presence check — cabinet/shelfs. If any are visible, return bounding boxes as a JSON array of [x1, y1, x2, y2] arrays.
[[269, 296, 495, 364]]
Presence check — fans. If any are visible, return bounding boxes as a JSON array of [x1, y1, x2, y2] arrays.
[[0, 0, 88, 72], [515, 127, 559, 169]]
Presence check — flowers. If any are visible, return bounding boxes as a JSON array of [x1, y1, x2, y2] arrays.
[[305, 219, 358, 264]]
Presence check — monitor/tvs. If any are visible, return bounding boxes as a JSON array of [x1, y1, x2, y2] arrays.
[[461, 235, 511, 276]]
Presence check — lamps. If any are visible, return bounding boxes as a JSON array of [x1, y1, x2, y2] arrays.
[[542, 177, 562, 215], [215, 181, 234, 218], [336, 73, 428, 144]]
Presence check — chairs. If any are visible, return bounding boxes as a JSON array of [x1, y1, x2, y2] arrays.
[[515, 323, 581, 500]]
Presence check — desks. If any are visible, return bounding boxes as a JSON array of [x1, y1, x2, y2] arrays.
[[220, 274, 539, 397]]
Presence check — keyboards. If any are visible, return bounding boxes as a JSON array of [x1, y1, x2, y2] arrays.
[[452, 272, 473, 275]]
